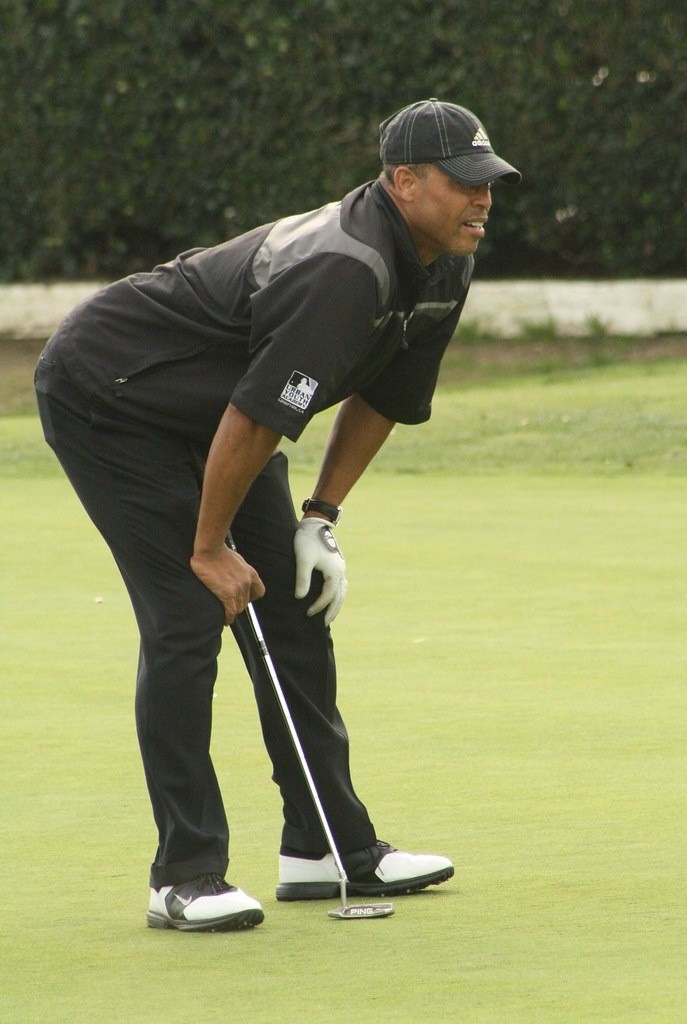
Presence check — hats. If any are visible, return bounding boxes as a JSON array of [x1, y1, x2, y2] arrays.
[[378, 97, 525, 189]]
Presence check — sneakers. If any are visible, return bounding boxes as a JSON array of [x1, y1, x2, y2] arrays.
[[276, 837, 455, 900], [146, 876, 267, 934]]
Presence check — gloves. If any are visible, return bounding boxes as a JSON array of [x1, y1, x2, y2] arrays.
[[294, 518, 350, 627]]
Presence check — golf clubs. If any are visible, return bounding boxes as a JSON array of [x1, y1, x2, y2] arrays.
[[190, 446, 394, 923]]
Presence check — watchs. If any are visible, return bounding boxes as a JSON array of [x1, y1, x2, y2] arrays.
[[303, 497, 342, 526]]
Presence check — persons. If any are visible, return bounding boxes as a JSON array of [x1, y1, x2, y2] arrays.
[[33, 96, 522, 933]]
